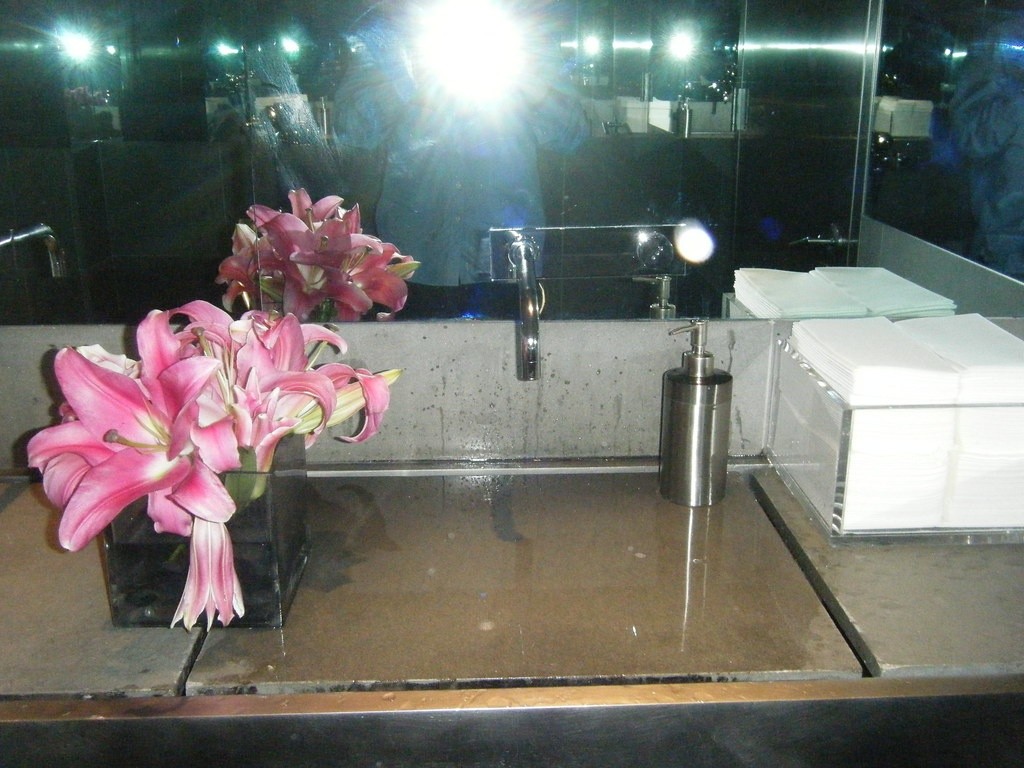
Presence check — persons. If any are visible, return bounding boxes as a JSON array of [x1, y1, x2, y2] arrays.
[[333, 2, 593, 323], [929, 19, 1023, 281]]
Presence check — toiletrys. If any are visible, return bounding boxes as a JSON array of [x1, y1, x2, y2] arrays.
[[631, 273, 678, 318], [657, 316, 734, 508]]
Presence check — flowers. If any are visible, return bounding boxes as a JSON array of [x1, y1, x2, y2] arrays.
[[63, 85, 109, 106], [214, 186, 422, 322], [25, 300, 401, 634]]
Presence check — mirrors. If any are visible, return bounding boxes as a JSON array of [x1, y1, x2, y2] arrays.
[[0, 0, 1024, 328]]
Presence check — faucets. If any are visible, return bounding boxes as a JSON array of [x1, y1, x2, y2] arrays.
[[512, 239, 544, 382]]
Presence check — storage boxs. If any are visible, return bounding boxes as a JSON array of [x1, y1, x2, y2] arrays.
[[764, 339, 1024, 548]]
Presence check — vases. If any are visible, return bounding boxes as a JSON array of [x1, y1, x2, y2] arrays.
[[101, 429, 311, 628], [66, 105, 124, 143]]
